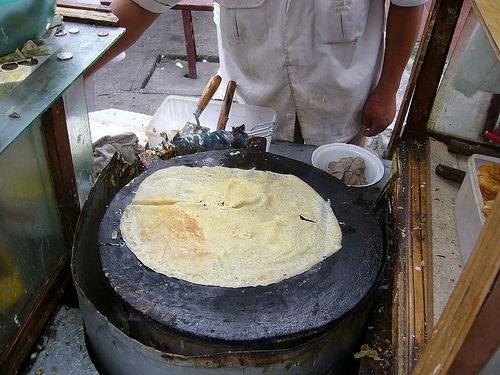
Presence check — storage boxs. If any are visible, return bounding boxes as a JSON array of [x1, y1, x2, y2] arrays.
[[144, 95, 277, 154], [454, 154, 500, 268]]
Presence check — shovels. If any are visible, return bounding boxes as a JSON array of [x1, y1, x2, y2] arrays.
[[178, 75, 223, 140]]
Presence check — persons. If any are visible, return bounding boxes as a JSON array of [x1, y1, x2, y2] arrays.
[[80, 0, 428, 147]]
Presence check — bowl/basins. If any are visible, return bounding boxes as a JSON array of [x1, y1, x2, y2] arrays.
[[311, 143, 386, 187]]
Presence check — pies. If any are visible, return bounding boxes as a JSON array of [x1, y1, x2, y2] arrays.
[[119, 162, 342, 289]]
